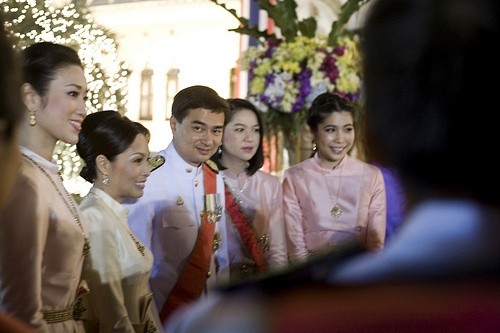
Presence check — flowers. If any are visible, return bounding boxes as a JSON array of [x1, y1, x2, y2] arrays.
[[209, 0, 371, 162]]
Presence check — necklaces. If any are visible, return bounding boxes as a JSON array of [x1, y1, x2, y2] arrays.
[[22, 154, 92, 257], [320, 162, 343, 218], [227, 177, 251, 199], [122, 225, 147, 256]]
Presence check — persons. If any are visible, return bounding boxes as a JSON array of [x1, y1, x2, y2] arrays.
[[76, 108, 163, 333], [124, 84, 232, 333], [210, 97, 288, 286], [281, 92, 387, 268], [160, 0, 500, 333], [0, 42, 88, 333]]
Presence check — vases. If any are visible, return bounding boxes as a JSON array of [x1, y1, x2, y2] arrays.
[[282, 122, 313, 166]]
